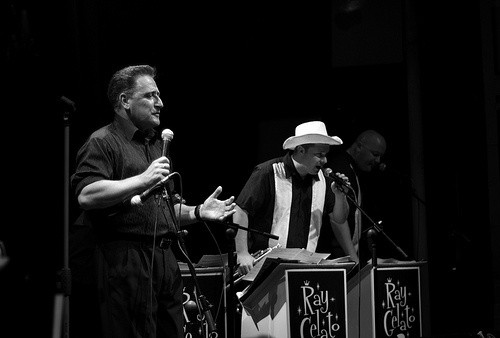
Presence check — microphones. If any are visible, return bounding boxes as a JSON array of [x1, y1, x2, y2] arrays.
[[161, 128, 173, 157], [131, 172, 175, 207], [324, 168, 349, 188], [379, 163, 396, 178]]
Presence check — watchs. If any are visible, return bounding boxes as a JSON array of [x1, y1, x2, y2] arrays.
[[194, 204, 204, 220]]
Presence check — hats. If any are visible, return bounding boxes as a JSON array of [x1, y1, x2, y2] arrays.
[[283, 121, 343, 150]]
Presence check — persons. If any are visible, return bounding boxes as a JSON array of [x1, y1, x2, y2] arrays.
[[233, 120, 351, 338], [70, 64, 237, 338], [325, 130, 387, 264]]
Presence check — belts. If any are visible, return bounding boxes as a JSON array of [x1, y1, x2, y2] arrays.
[[127, 234, 174, 249]]
